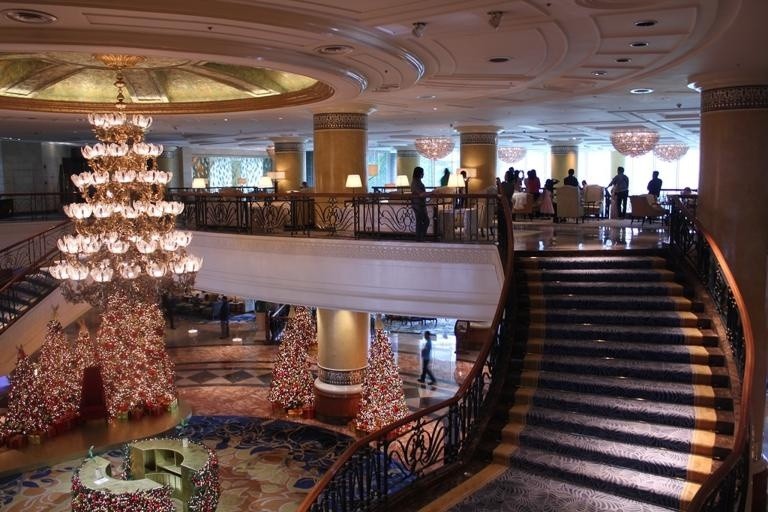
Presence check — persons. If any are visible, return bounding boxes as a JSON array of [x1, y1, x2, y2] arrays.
[[646, 171, 662, 198], [501, 166, 587, 223], [416, 331, 436, 385], [409, 166, 441, 242], [681, 187, 695, 205], [160, 291, 176, 329], [264, 303, 289, 342], [460, 171, 467, 181], [439, 168, 450, 186], [216, 295, 229, 339], [189, 293, 211, 311], [603, 166, 628, 219]]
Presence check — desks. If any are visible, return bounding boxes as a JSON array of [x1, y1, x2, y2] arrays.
[[79, 455, 164, 496], [129, 438, 210, 512]]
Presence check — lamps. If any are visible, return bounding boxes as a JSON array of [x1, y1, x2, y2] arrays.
[[415, 138, 455, 161], [345, 174, 363, 207], [655, 144, 690, 163], [498, 147, 527, 164], [610, 127, 659, 158], [257, 176, 273, 206], [268, 172, 285, 198], [395, 174, 410, 193], [192, 177, 206, 192], [48, 54, 205, 310], [447, 173, 466, 209], [457, 168, 476, 193], [368, 165, 379, 181]]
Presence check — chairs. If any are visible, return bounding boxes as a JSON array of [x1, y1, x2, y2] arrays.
[[435, 184, 669, 237]]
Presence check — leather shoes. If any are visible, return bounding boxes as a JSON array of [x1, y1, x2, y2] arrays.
[[417, 379, 437, 385]]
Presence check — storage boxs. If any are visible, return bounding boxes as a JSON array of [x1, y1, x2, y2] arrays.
[[0, 420, 68, 449], [117, 397, 178, 420], [272, 401, 315, 419]]
